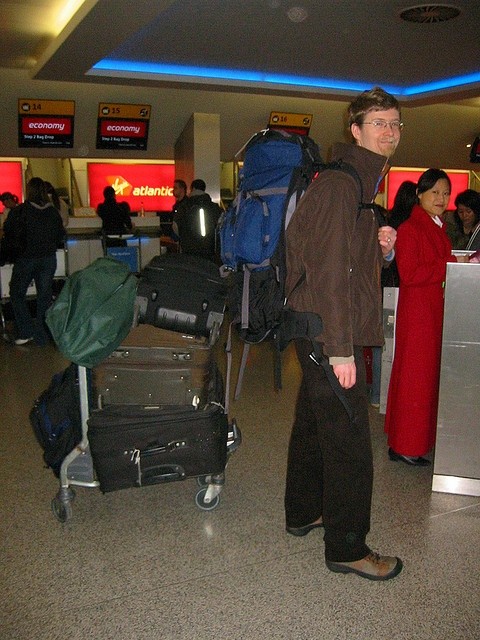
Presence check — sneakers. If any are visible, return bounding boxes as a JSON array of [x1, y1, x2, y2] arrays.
[[285, 517, 323, 536], [326, 551, 402, 581]]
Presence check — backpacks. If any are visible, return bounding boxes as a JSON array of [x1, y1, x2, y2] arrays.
[[217, 126, 321, 268]]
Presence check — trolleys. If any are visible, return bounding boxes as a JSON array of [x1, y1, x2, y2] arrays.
[[50, 364, 232, 524]]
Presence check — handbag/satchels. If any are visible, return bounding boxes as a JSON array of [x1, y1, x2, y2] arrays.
[[29, 360, 92, 477], [224, 258, 288, 352], [46, 255, 140, 372]]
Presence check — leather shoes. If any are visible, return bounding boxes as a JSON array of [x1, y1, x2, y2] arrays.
[[388, 448, 431, 467]]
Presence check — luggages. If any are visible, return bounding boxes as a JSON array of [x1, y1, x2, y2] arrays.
[[87, 400, 241, 492], [136, 250, 229, 337], [94, 322, 217, 407]]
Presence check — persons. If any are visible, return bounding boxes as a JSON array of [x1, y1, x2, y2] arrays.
[[370, 181, 418, 409], [170, 177, 188, 254], [383, 168, 470, 467], [455, 188, 480, 249], [283, 90, 402, 581], [2, 192, 18, 264], [172, 181, 221, 264], [97, 187, 133, 274], [44, 182, 70, 232], [7, 176, 65, 348]]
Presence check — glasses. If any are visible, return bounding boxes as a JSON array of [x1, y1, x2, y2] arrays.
[[363, 118, 405, 131]]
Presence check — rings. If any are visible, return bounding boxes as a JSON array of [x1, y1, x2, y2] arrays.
[[387, 237, 393, 244]]
